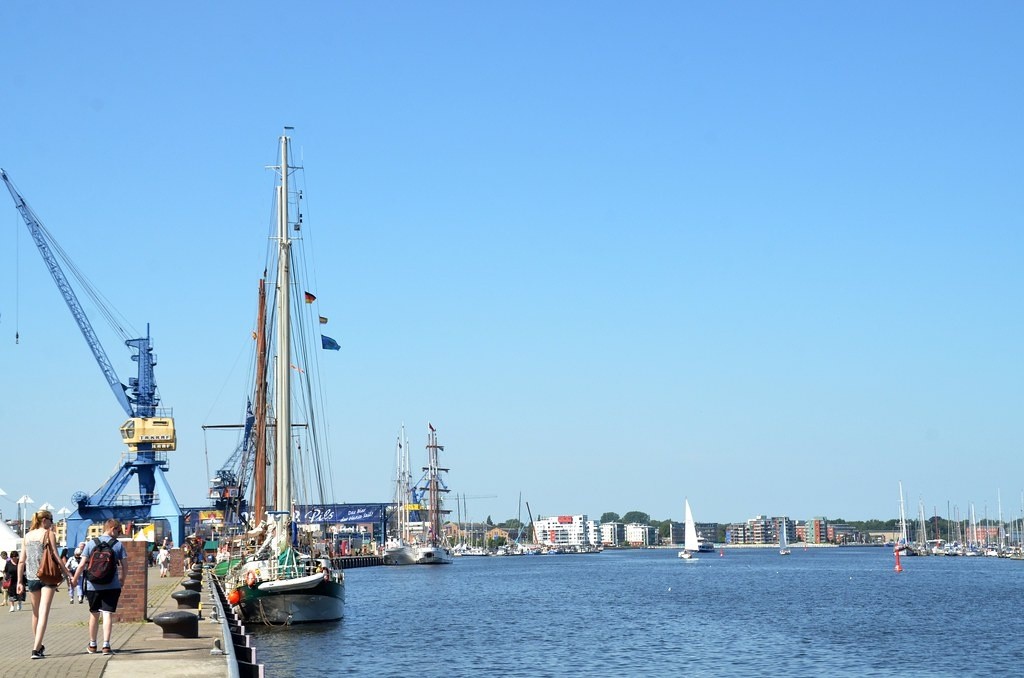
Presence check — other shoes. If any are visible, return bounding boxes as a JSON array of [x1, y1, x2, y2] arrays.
[[0, 601, 8, 606], [78, 597, 83, 604], [69, 599, 74, 605]]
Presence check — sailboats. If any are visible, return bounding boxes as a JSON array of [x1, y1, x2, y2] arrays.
[[678, 499, 701, 559], [913, 487, 1024, 560], [894, 480, 915, 557], [384, 422, 528, 562], [780, 516, 791, 555], [211, 126, 348, 633]]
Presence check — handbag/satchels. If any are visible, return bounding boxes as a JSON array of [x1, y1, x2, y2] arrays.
[[36, 529, 64, 586], [2, 577, 12, 589]]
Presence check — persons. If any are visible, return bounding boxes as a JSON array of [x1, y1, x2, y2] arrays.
[[148, 536, 205, 578], [357, 546, 362, 556], [71, 518, 129, 655], [17, 510, 72, 659], [0, 543, 92, 613]]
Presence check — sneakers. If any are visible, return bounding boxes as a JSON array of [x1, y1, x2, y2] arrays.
[[9, 606, 15, 613], [101, 646, 114, 655], [31, 644, 46, 659], [86, 643, 98, 653], [16, 602, 22, 610]]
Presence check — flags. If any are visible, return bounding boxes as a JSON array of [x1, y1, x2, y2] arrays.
[[304, 292, 316, 304], [319, 316, 327, 324], [321, 335, 341, 351]]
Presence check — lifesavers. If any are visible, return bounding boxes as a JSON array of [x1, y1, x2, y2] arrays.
[[246, 569, 255, 586], [320, 567, 329, 581]]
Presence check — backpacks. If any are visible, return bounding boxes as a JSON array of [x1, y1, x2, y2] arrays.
[[83, 536, 119, 586]]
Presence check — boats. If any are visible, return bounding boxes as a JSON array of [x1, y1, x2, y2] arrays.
[[529, 545, 604, 554], [886, 541, 898, 546], [696, 532, 715, 553]]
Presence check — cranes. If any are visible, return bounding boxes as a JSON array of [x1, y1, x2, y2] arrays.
[[203, 404, 259, 530], [0, 167, 176, 524]]
